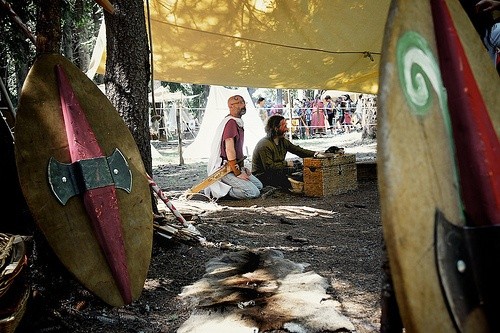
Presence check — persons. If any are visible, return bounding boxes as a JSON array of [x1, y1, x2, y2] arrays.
[[197, 94, 265, 200], [459, 0, 500, 75], [251, 115, 328, 189], [254, 92, 377, 140]]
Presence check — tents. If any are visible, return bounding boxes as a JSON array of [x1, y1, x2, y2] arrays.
[[150, 86, 202, 139], [87, 0, 394, 95], [182, 85, 270, 163]]
